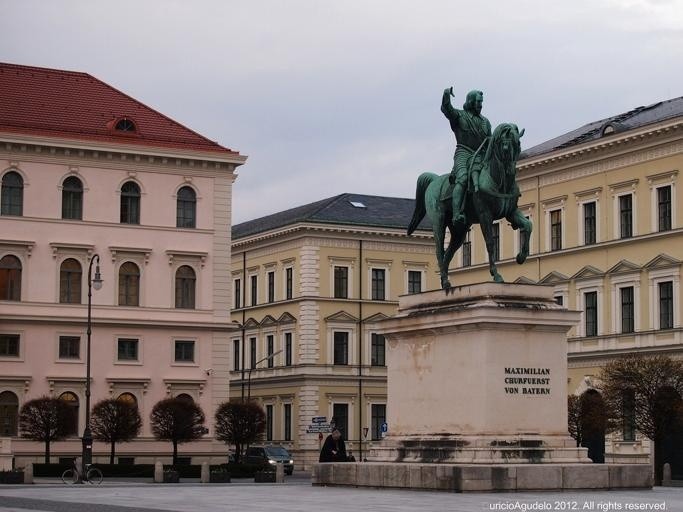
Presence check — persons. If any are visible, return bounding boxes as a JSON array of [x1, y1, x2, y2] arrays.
[[319, 430, 341, 462], [441, 86, 491, 228]]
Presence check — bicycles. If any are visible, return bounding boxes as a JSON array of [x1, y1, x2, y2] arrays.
[[62, 464, 104, 485]]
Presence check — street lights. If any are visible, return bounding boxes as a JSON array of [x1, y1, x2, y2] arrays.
[[245, 348, 290, 464], [81, 252, 103, 484]]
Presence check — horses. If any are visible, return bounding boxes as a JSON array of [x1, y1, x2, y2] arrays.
[[407, 123, 533, 290]]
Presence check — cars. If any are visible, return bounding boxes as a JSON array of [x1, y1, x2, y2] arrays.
[[247, 446, 295, 477]]
